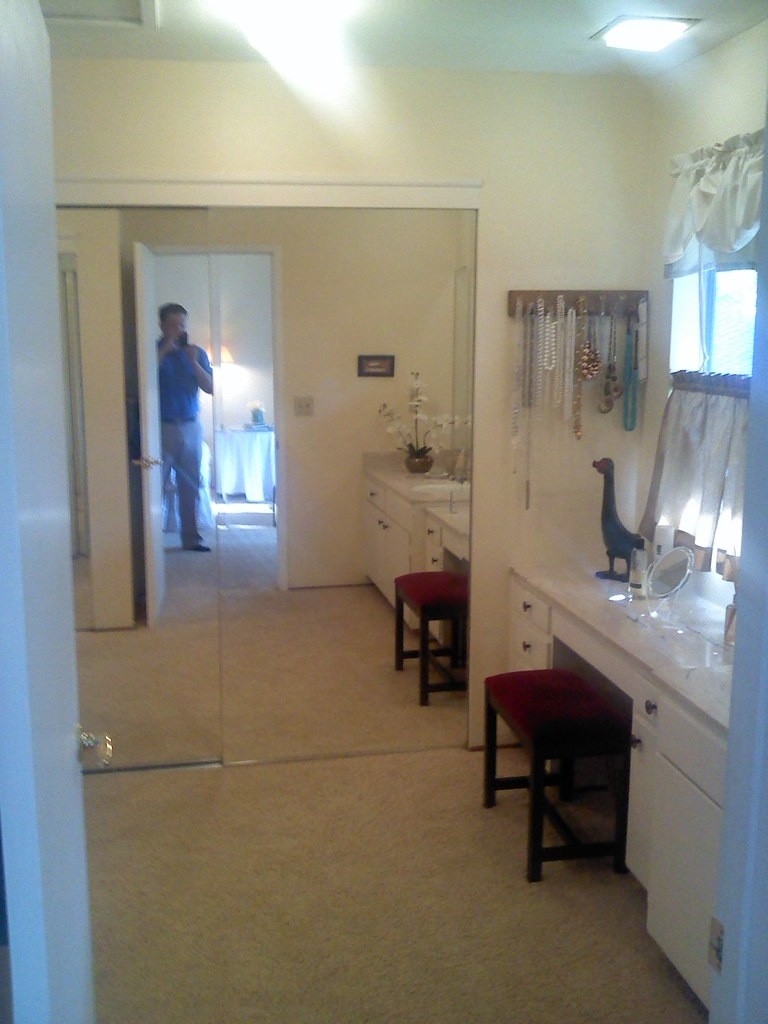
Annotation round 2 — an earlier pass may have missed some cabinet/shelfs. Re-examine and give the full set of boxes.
[361,469,472,628]
[423,506,470,643]
[645,664,733,1009]
[508,565,736,888]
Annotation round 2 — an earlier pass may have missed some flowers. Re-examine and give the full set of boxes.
[377,371,472,455]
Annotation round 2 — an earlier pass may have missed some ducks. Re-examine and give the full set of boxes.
[593,458,646,583]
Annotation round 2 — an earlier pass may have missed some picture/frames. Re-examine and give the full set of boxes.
[357,355,395,378]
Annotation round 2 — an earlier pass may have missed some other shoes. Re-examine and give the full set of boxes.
[190,544,210,552]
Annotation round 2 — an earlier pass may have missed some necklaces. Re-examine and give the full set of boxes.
[512,293,650,508]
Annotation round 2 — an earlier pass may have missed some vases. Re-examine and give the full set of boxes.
[404,455,432,473]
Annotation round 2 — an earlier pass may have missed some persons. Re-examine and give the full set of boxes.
[158,303,214,552]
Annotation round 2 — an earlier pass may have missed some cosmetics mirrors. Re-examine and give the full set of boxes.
[646,545,694,599]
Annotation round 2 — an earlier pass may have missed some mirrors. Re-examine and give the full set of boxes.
[642,547,694,622]
[57,207,478,769]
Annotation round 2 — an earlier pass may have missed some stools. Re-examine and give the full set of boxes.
[481,667,631,883]
[393,572,471,706]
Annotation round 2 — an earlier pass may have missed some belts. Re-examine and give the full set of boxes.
[162,416,198,423]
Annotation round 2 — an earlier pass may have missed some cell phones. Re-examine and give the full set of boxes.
[178,332,188,352]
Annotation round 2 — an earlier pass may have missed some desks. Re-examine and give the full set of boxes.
[215,428,275,504]
